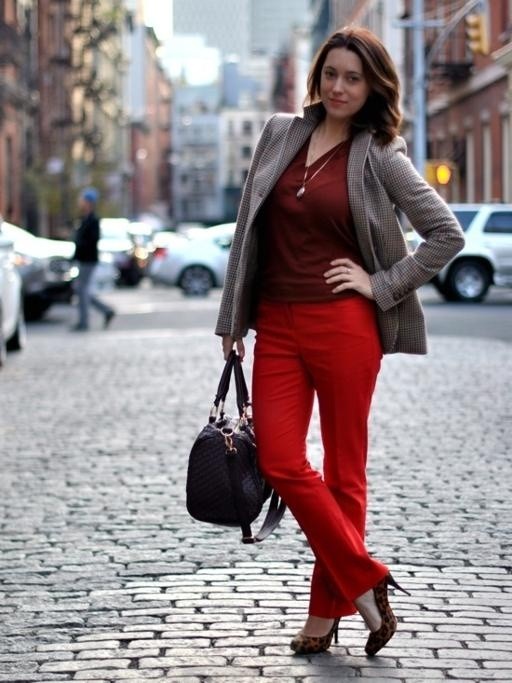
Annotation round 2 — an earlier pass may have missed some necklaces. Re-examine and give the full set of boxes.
[296,125,343,199]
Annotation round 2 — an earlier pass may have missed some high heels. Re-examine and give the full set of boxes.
[290,616,340,655]
[364,570,411,658]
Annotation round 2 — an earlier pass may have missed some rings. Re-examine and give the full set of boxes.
[347,267,351,274]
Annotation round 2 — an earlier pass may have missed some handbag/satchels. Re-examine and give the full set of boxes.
[185,416,272,528]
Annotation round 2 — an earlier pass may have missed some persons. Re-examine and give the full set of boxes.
[215,22,468,658]
[65,185,115,331]
